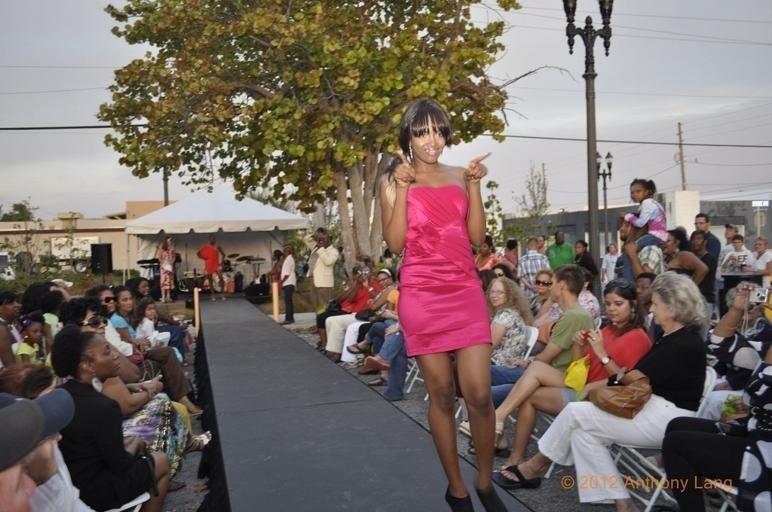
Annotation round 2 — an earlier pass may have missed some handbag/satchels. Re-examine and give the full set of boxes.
[588,369,652,418]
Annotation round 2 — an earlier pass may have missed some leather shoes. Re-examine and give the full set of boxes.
[446,485,474,511]
[368,377,386,385]
[360,366,378,374]
[168,479,185,491]
[459,418,504,447]
[476,484,508,511]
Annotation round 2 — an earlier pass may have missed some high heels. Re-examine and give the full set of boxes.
[183,430,212,460]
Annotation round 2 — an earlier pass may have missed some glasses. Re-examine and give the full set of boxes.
[78,315,108,328]
[100,296,119,303]
[535,279,552,286]
[606,280,630,289]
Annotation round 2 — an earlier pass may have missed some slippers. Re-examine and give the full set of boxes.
[189,408,203,415]
[347,343,370,353]
[365,356,391,370]
[491,464,541,490]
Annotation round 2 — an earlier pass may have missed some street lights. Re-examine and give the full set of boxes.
[593,151,612,255]
[563,1,614,309]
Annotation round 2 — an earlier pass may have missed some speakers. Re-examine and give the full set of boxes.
[245,283,269,305]
[91,243,112,274]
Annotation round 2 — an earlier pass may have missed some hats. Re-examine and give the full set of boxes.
[0,388,74,473]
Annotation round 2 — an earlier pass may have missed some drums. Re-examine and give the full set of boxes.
[222,260,230,270]
[211,271,235,292]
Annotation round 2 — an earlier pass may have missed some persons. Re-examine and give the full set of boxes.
[200,236,226,301]
[269,227,407,403]
[451,178,772,512]
[379,97,506,511]
[158,236,176,302]
[0,278,214,508]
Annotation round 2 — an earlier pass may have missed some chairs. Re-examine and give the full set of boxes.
[402,316,772,512]
[103,491,151,512]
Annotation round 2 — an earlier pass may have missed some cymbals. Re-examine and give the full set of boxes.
[228,254,240,258]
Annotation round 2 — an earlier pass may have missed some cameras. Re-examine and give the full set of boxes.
[357,270,368,279]
[749,288,768,303]
